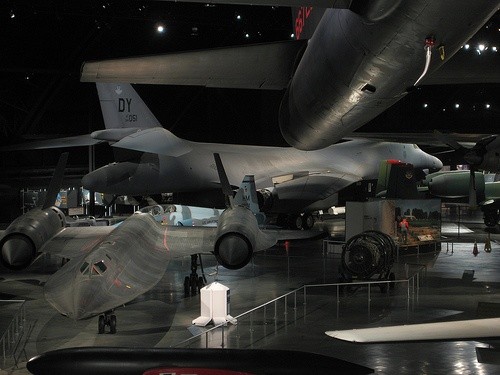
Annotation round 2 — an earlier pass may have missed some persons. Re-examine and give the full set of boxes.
[398,217,410,244]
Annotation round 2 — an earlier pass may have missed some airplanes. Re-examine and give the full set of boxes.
[1,1,497,344]
